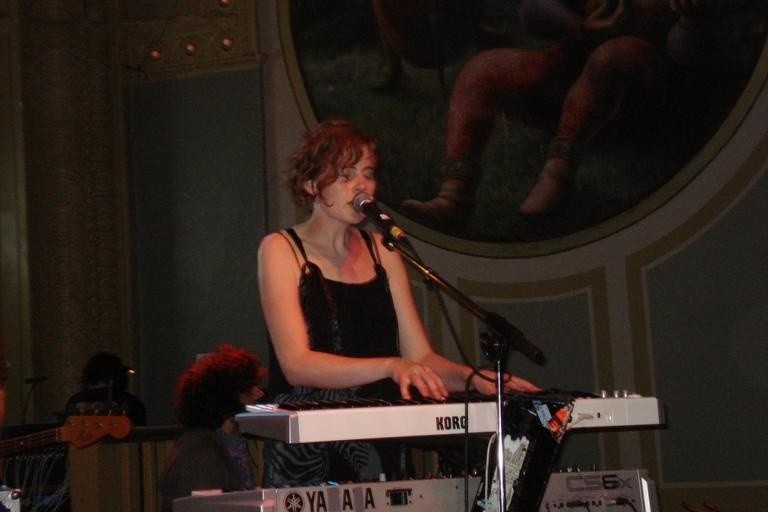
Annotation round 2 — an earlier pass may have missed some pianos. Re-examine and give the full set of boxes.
[172,390,669,512]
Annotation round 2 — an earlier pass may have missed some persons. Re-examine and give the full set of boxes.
[64,353,147,427]
[398,1,709,217]
[155,340,271,511]
[256,119,545,485]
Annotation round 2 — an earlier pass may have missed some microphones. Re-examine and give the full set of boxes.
[25,376,49,384]
[353,192,410,244]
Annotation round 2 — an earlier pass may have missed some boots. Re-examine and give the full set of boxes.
[515,134,591,224]
[399,155,481,235]
